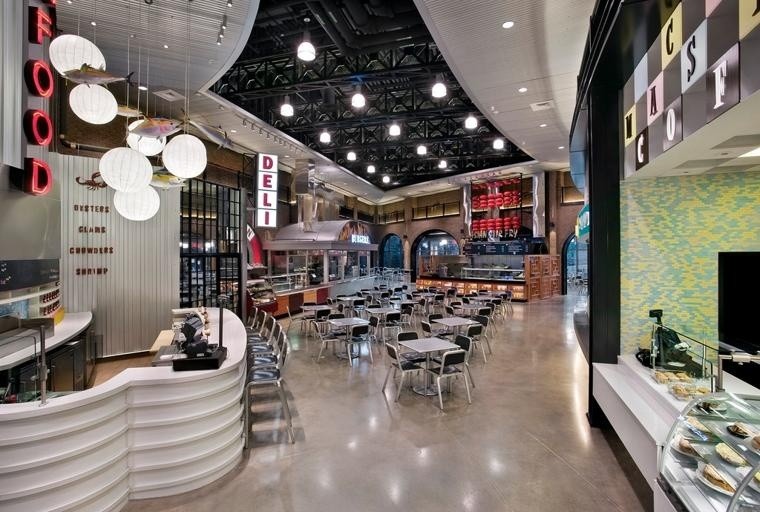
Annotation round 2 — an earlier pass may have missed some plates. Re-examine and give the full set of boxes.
[670,438,697,458]
[694,467,735,497]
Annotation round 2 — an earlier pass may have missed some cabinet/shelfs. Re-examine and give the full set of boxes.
[0,321,98,393]
[656,392,760,512]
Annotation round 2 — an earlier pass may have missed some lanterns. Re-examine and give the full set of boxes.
[472,179,520,231]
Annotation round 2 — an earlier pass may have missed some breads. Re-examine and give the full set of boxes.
[679,416,760,492]
[656,372,709,398]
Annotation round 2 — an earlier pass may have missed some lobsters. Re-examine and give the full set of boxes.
[76,171,107,191]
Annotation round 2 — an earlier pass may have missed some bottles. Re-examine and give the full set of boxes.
[43,290,59,315]
[205,318,210,328]
[204,308,208,318]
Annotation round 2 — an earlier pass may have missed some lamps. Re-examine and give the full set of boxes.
[279,29,506,184]
[48,0,207,220]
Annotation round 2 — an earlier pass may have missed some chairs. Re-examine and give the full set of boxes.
[239,266,512,449]
